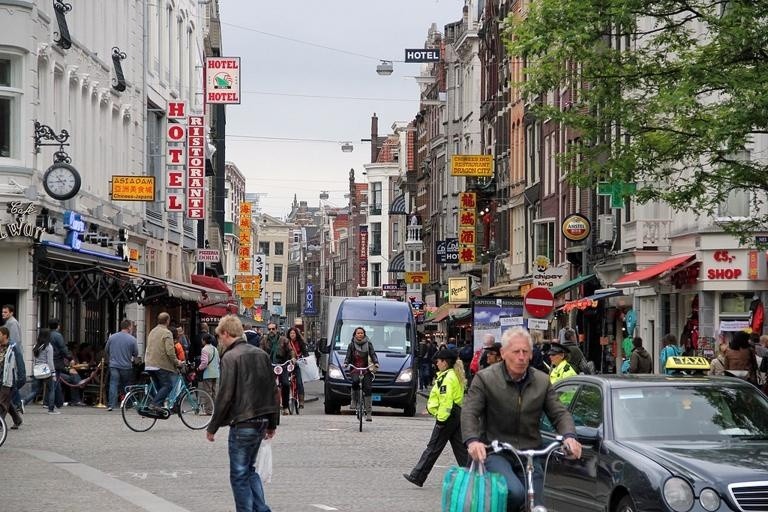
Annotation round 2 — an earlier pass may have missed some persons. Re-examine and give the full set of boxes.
[343,327,380,421]
[402,349,468,488]
[105,319,138,411]
[170,321,308,416]
[419,330,590,404]
[460,324,583,512]
[706,330,768,393]
[2,304,26,415]
[144,311,183,413]
[206,314,280,512]
[0,327,26,429]
[629,337,652,374]
[21,317,105,415]
[660,334,682,375]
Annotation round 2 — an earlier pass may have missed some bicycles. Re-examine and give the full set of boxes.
[468,432,591,512]
[0,415,7,448]
[120,361,215,433]
[341,359,379,432]
[287,357,304,416]
[271,359,292,425]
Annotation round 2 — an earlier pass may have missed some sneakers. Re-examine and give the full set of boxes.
[148,404,164,413]
[48,410,61,415]
[21,400,24,414]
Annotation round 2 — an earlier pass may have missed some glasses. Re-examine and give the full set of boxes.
[268,327,277,331]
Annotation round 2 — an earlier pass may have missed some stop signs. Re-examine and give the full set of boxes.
[524,286,556,318]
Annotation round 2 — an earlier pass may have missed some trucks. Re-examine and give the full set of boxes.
[313,295,391,382]
[318,298,421,416]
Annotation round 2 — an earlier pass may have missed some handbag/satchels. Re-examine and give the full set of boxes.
[367,355,377,373]
[440,458,508,511]
[33,364,52,379]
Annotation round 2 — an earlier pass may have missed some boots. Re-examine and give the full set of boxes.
[298,394,304,408]
[350,388,358,409]
[364,396,373,421]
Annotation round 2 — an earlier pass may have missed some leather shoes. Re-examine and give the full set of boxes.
[403,474,423,487]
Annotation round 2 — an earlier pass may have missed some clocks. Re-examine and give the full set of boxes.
[42,162,81,200]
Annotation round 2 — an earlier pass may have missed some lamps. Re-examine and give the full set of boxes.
[319,189,330,199]
[342,141,352,153]
[376,59,394,76]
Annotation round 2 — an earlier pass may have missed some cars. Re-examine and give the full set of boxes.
[528,356,767,512]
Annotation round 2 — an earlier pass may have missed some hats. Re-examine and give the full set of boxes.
[484,343,501,354]
[547,342,571,355]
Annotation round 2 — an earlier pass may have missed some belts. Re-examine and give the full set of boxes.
[231,422,268,429]
